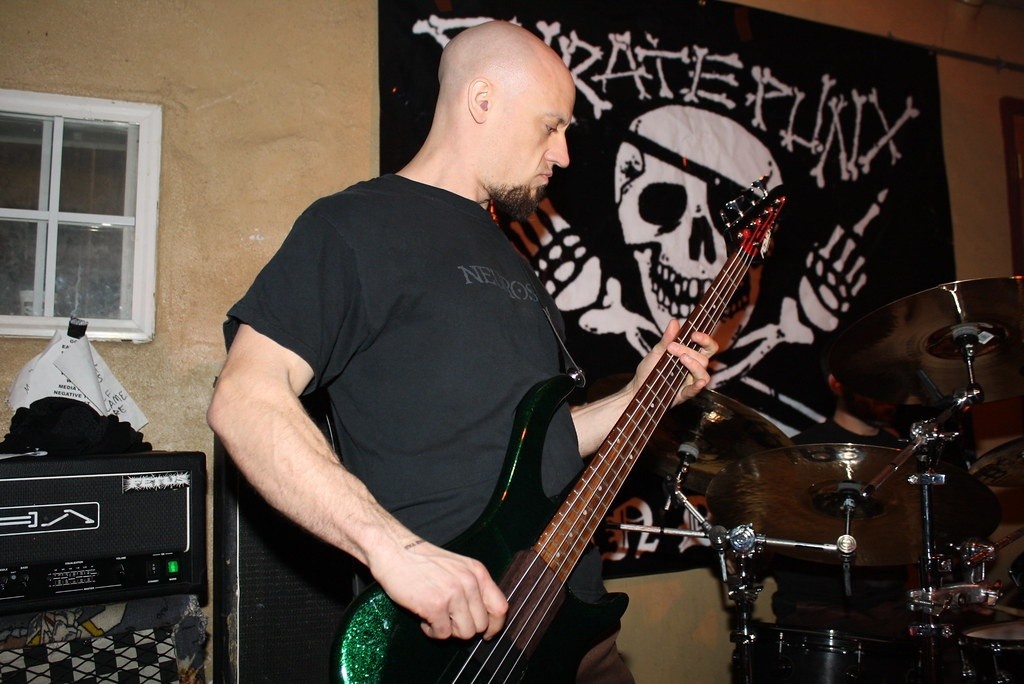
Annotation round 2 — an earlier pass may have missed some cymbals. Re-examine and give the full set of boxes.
[968,434,1024,489]
[645,385,796,476]
[705,442,1002,569]
[832,275,1023,407]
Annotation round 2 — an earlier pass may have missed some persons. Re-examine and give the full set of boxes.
[208,21,719,684]
[773,323,924,640]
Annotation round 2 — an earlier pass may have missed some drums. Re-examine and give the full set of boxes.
[732,620,931,684]
[957,616,1024,684]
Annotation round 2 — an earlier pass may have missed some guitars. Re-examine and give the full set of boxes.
[326,177,788,684]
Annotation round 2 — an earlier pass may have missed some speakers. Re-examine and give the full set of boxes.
[212,434,360,684]
